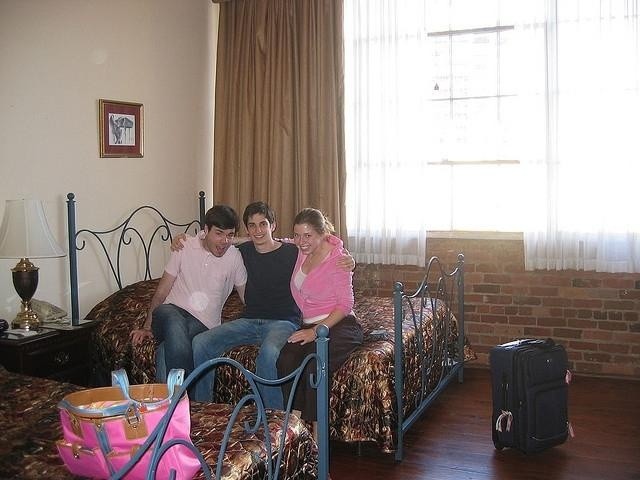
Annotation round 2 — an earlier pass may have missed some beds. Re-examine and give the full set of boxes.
[0,325,331,480]
[66,192,465,462]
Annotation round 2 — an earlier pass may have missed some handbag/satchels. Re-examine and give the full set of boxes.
[55,368,202,480]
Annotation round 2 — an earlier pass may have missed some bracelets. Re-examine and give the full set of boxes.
[312,327,317,338]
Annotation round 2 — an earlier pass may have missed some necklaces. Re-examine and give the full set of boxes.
[309,256,312,265]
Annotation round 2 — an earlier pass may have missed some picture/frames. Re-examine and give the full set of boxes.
[99,99,144,158]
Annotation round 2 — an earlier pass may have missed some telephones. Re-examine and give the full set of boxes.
[20,298,67,320]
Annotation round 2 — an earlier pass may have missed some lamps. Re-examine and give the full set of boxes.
[0,199,67,331]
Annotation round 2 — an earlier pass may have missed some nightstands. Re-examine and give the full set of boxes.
[0,320,101,387]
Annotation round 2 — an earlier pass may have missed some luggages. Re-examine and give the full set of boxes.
[488,336,571,456]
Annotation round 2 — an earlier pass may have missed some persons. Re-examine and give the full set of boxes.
[128,205,247,401]
[230,208,363,443]
[170,202,356,413]
